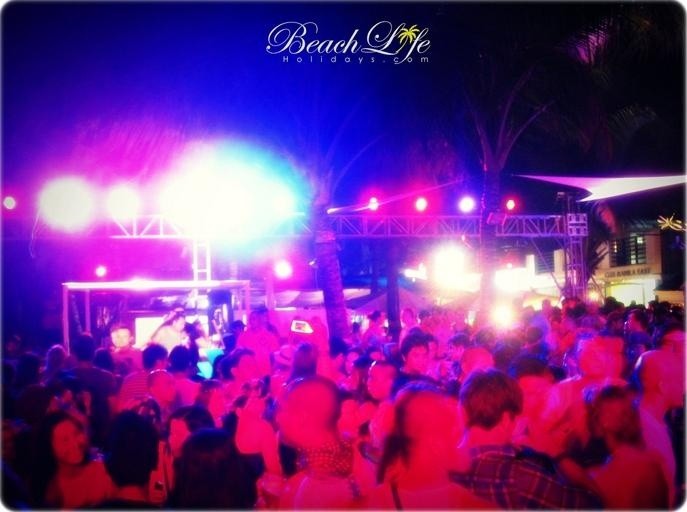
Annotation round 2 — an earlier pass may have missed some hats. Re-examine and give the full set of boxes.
[273,344,296,367]
